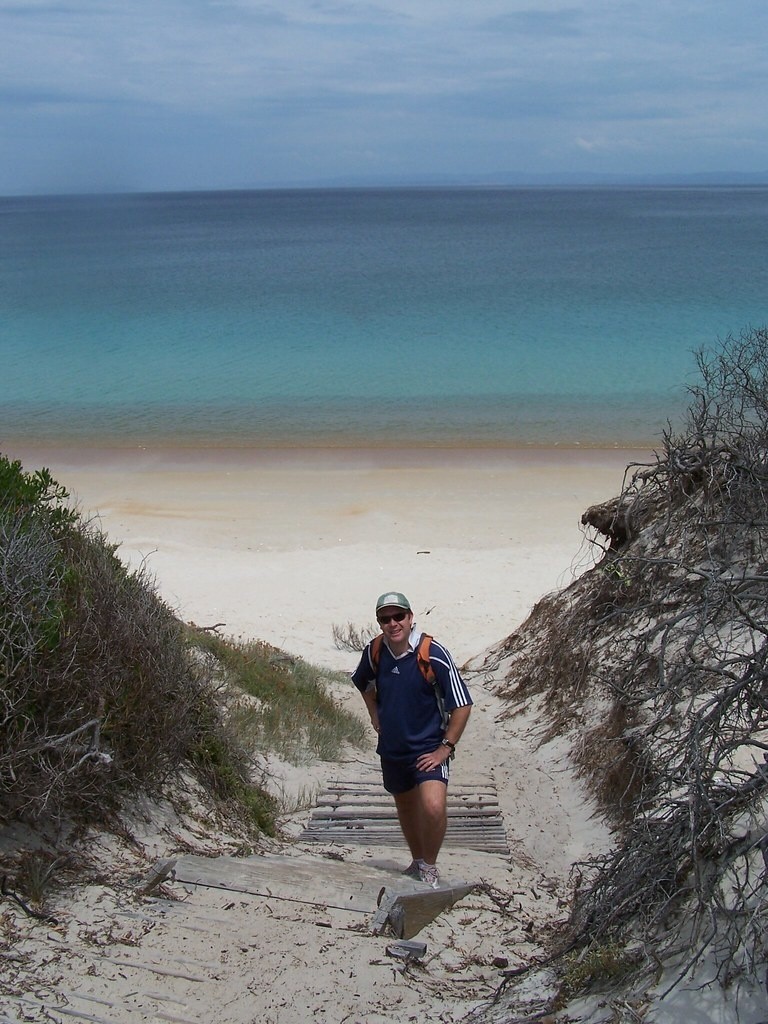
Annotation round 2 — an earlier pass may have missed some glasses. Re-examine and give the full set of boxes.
[377,613,406,624]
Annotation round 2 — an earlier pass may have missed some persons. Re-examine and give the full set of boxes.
[350,592,473,890]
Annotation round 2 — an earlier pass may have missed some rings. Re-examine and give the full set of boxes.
[429,761,432,764]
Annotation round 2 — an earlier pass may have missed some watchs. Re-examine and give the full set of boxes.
[441,738,454,749]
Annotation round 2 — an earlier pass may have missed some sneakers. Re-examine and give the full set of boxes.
[401,861,419,877]
[418,864,441,890]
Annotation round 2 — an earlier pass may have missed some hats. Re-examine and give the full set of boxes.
[376,592,410,612]
